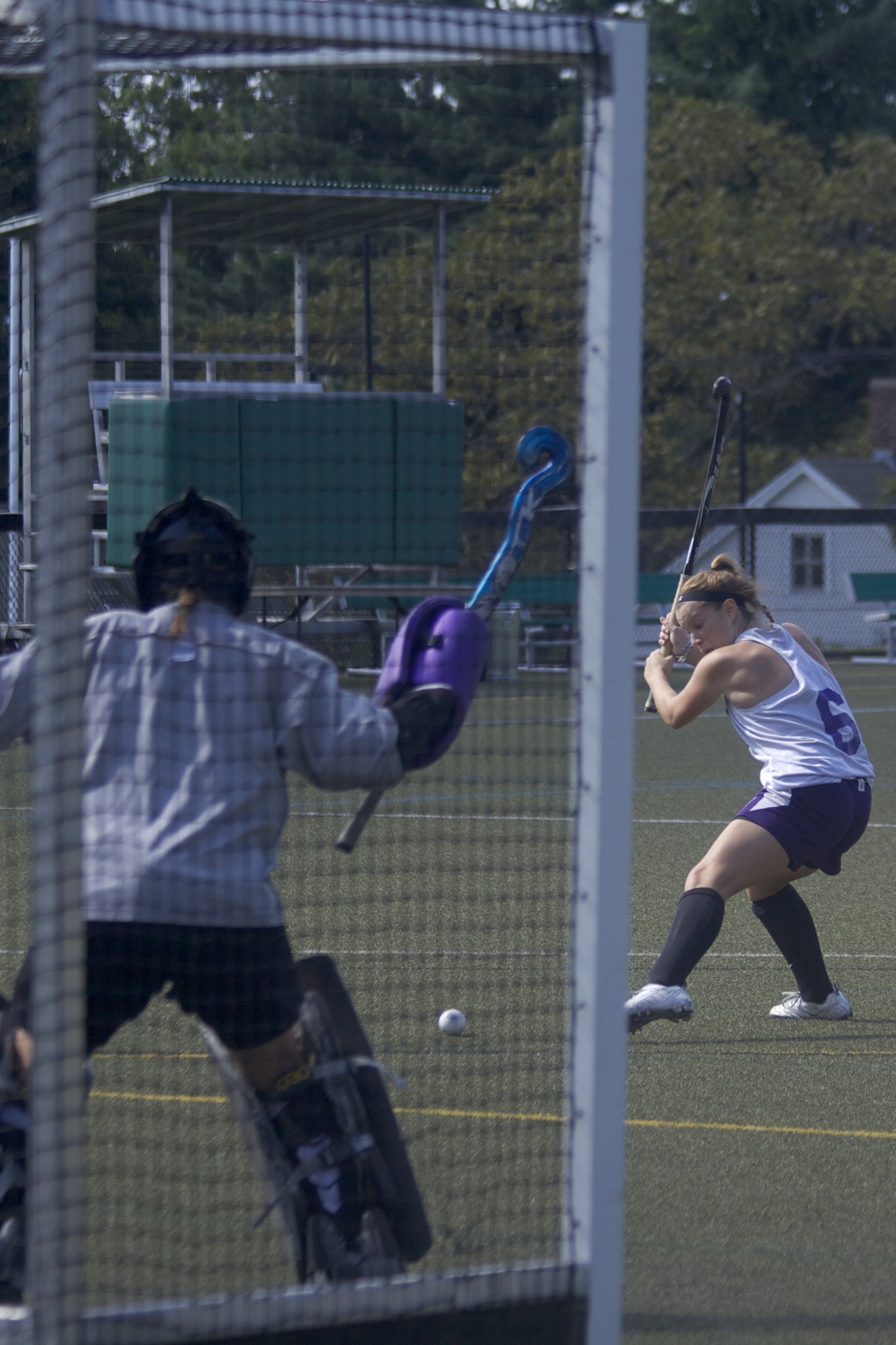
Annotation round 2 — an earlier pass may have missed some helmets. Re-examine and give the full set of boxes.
[133,485,253,619]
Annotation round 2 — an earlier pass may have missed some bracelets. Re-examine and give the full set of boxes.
[676,640,693,664]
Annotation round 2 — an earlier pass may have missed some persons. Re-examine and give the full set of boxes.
[626,552,875,1036]
[0,491,494,1307]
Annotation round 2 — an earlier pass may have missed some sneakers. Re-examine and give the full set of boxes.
[624,983,694,1034]
[768,983,854,1022]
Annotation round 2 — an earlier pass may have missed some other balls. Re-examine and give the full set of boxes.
[438,1008,466,1035]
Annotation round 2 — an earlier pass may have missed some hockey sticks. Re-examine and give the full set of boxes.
[641,375,734,715]
[334,426,576,856]
